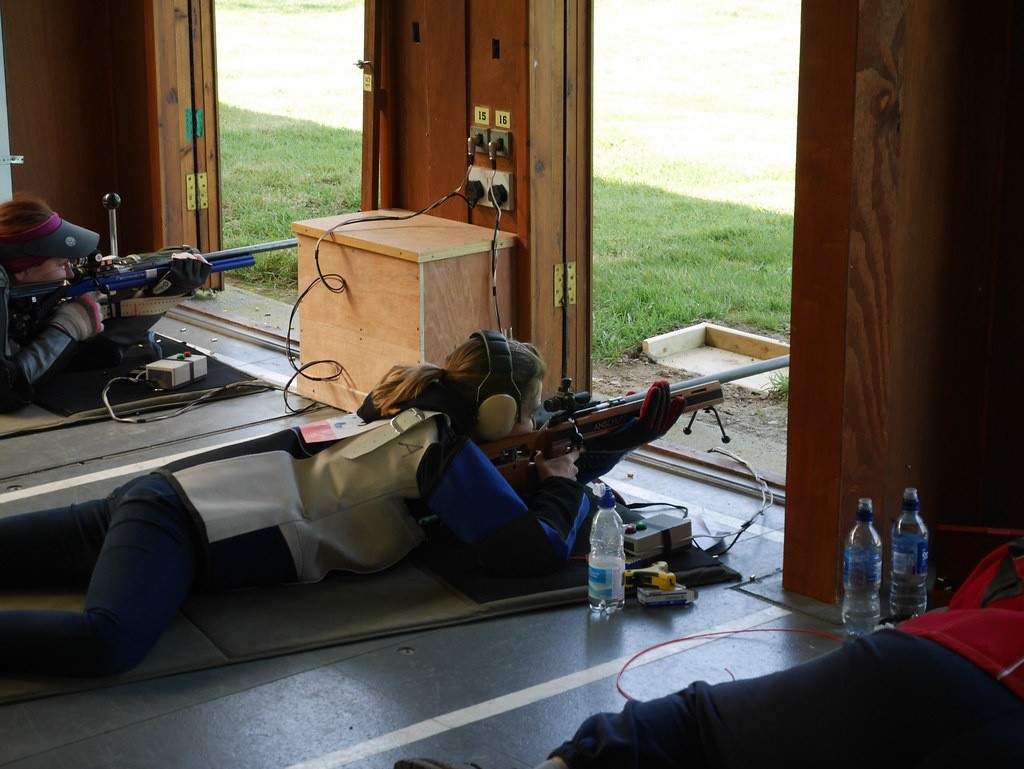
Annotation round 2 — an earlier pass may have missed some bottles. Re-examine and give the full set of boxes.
[587,488,625,614]
[888,487,930,619]
[841,498,883,635]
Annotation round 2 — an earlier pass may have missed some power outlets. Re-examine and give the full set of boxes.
[466,165,513,211]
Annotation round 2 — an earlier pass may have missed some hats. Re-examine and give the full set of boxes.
[0,212,100,274]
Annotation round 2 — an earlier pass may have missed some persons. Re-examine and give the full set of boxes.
[0,329,688,680]
[0,198,212,414]
[393,540,1024,769]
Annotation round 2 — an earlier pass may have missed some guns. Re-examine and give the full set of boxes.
[5,237,299,311]
[476,353,791,497]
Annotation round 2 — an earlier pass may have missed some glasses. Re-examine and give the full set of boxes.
[28,259,70,275]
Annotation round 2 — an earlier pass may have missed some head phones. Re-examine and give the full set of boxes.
[468,330,522,439]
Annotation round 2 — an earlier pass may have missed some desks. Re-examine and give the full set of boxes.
[292,208,520,416]
